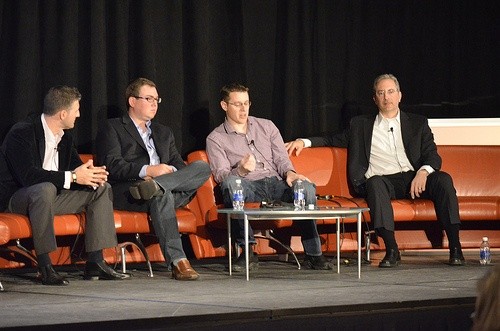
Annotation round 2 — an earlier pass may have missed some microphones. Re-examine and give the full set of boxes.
[58,147,61,152]
[391,127,393,132]
[251,140,254,144]
[149,134,153,139]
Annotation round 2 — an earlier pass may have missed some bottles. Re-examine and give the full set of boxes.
[293,178,306,208]
[232,179,244,210]
[479,237,491,264]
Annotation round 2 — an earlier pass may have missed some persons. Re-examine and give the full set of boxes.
[96,77,212,280]
[206,84,331,272]
[0,85,130,285]
[285,75,466,266]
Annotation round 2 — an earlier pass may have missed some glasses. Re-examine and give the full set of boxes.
[135,95,162,104]
[377,88,398,97]
[228,101,252,108]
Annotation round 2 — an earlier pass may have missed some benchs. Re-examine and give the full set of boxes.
[0,153,198,291]
[186,143,499,260]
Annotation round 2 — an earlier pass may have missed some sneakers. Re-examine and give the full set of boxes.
[303,256,334,271]
[232,252,260,272]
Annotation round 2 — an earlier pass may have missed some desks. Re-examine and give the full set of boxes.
[218,207,372,280]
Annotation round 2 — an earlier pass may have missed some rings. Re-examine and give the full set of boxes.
[418,188,422,189]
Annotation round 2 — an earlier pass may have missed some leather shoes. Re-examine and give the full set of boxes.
[36,264,69,285]
[83,259,132,281]
[129,177,158,200]
[172,259,200,280]
[379,248,401,268]
[449,245,465,265]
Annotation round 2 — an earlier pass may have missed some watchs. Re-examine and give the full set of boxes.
[72,171,77,182]
